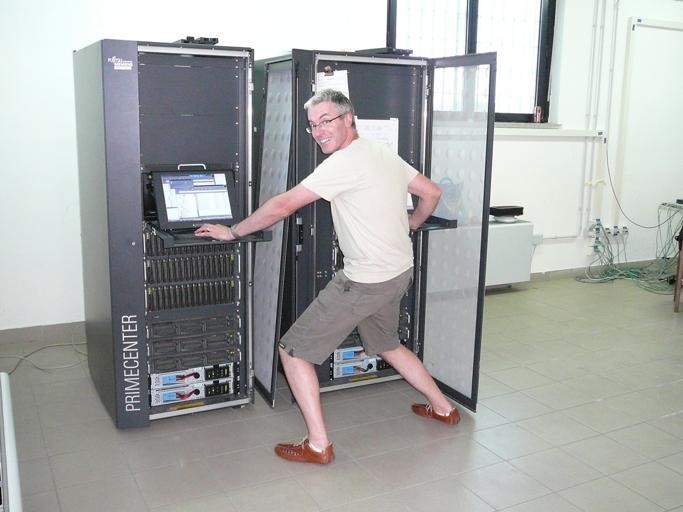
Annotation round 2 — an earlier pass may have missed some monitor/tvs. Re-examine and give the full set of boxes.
[151,171,239,231]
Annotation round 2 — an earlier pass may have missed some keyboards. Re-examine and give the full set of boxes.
[174,231,257,244]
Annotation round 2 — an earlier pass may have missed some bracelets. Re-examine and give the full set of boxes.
[230,224,240,239]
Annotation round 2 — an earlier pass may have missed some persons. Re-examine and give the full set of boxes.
[193,88,461,466]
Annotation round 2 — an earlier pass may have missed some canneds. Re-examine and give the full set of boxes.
[534,106,542,123]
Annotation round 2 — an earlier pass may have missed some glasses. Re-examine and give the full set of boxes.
[304,114,344,135]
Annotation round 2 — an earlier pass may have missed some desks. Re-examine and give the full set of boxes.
[660,198,681,313]
[426,218,534,295]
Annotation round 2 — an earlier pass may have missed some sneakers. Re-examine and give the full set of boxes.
[274,435,336,466]
[411,400,461,426]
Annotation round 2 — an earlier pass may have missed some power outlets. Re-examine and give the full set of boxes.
[587,218,630,258]
